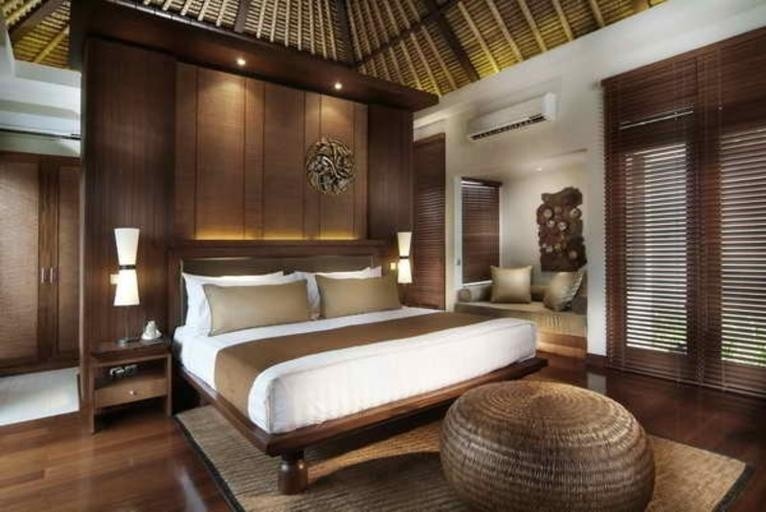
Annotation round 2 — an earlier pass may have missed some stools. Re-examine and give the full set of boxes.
[437,377,657,510]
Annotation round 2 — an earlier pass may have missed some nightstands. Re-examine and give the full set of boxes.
[81,333,174,435]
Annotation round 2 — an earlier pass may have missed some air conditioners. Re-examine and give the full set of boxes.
[466,91,557,142]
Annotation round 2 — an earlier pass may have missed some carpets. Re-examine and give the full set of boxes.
[170,398,752,512]
[0,362,82,428]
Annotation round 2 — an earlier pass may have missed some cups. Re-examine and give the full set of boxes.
[145,320,156,336]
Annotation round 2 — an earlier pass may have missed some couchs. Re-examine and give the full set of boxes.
[454,280,587,360]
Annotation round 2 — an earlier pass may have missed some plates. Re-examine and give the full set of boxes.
[140,331,163,341]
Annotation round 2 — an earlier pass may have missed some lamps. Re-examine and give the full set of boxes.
[389,231,414,285]
[107,226,140,307]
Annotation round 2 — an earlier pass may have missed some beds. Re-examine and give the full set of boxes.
[161,235,549,496]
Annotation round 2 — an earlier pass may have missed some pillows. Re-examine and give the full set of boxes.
[177,264,402,337]
[489,264,534,304]
[542,269,586,312]
